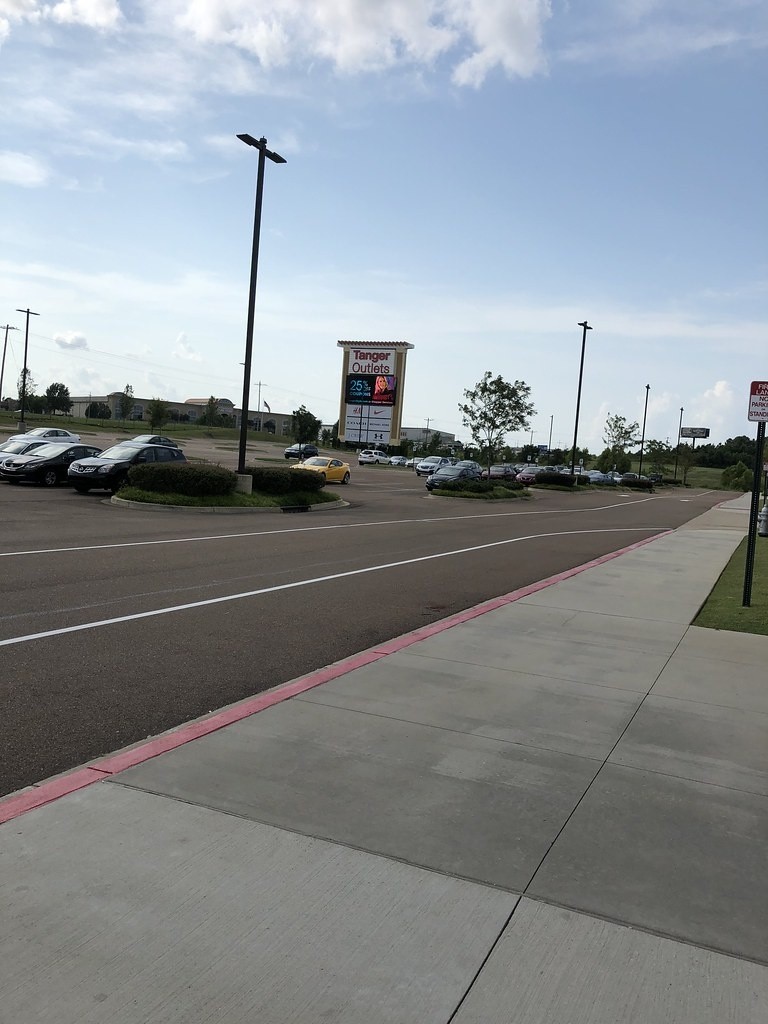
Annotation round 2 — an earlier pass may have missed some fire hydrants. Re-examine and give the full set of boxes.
[757,504,768,536]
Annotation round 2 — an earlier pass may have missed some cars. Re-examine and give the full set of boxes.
[482,465,517,481]
[391,456,409,467]
[15,410,29,414]
[0,439,54,463]
[130,435,178,448]
[289,456,351,484]
[447,457,460,465]
[0,442,105,487]
[8,426,81,444]
[455,460,483,475]
[514,464,650,485]
[426,465,480,490]
[515,466,548,486]
[404,457,423,467]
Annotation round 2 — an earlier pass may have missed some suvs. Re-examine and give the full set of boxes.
[415,455,452,476]
[284,443,319,460]
[358,450,392,467]
[67,440,189,495]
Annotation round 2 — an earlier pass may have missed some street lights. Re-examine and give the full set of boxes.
[548,415,553,458]
[16,308,41,422]
[572,321,593,477]
[639,384,650,480]
[255,384,267,430]
[675,407,684,479]
[235,132,286,474]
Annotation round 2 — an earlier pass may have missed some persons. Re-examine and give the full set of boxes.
[374,376,388,393]
